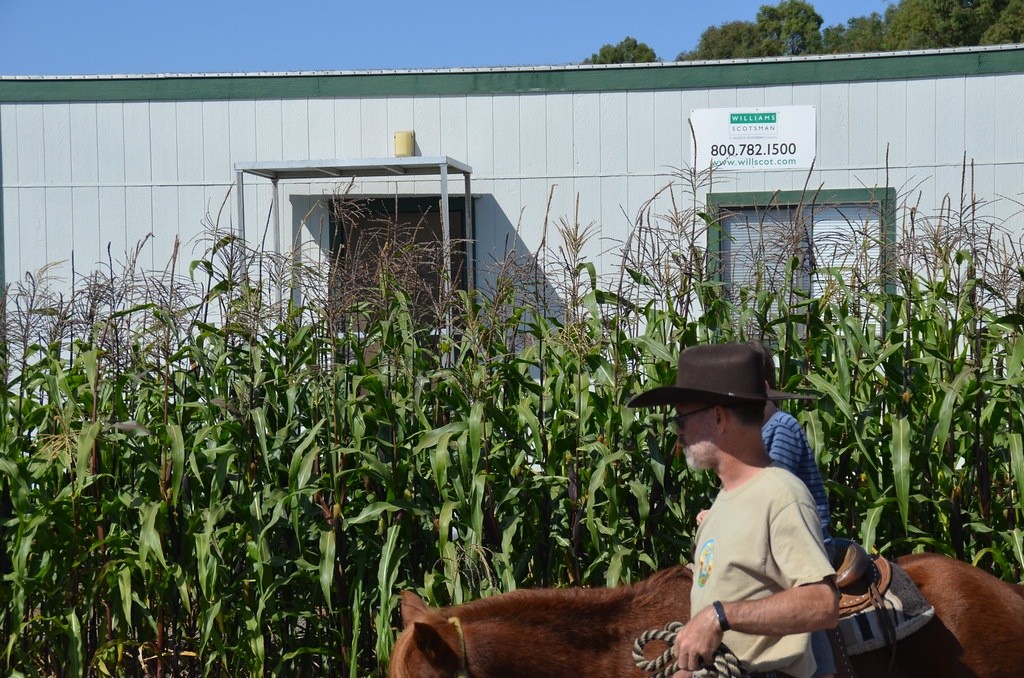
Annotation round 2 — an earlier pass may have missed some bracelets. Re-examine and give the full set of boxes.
[712,600,730,632]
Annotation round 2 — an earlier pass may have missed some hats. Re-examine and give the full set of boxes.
[627,344,818,407]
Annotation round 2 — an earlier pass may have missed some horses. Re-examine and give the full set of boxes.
[386,550,1024,678]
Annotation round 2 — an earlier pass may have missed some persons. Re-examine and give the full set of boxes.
[696,341,838,678]
[628,343,840,678]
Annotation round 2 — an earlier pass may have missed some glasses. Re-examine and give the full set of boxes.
[671,406,711,428]
